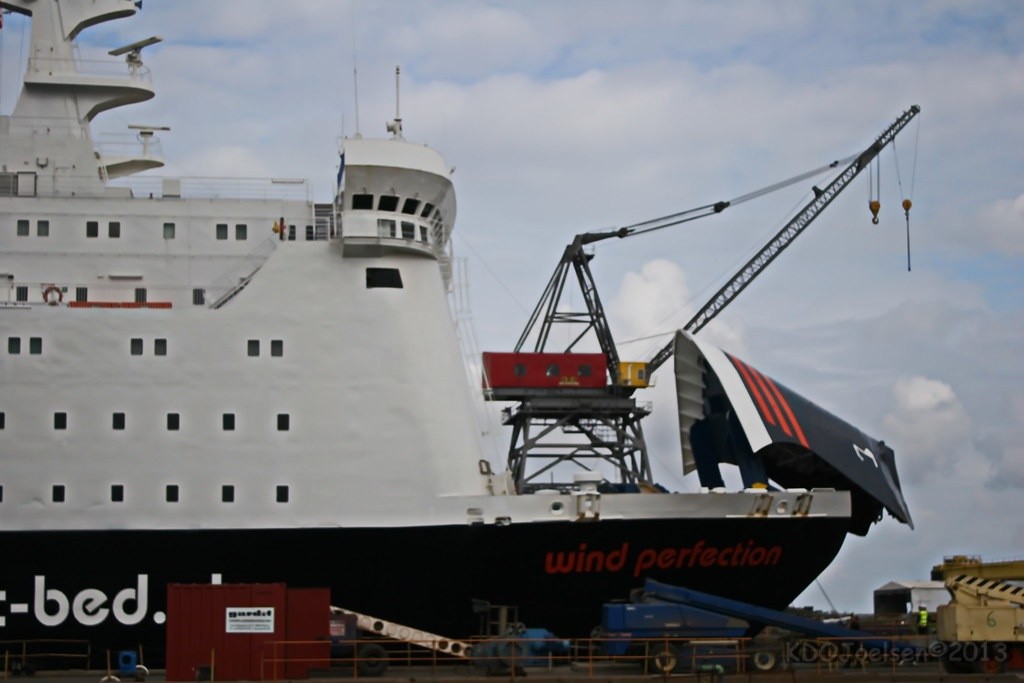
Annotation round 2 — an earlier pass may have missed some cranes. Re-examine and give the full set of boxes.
[482,86,923,499]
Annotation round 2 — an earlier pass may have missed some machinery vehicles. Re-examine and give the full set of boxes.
[923,554,1024,673]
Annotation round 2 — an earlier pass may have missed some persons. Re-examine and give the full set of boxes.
[917,604,928,635]
[846,614,860,630]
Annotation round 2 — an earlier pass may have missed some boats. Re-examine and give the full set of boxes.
[0,0,856,641]
